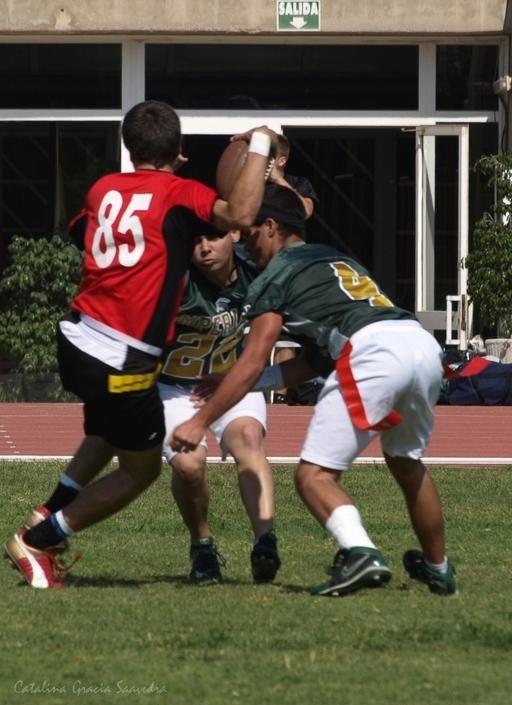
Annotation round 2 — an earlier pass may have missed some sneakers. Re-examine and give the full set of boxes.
[186,538,222,583]
[2,505,65,589]
[311,548,393,597]
[403,548,458,597]
[251,533,281,584]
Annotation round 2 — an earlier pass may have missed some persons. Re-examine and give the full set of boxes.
[269,134,320,403]
[170,187,456,598]
[157,223,280,584]
[3,100,279,590]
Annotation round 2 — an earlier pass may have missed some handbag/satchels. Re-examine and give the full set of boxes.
[438,344,512,404]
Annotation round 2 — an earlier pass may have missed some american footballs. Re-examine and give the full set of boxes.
[215,139,276,201]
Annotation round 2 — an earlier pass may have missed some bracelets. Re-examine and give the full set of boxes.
[248,130,271,157]
